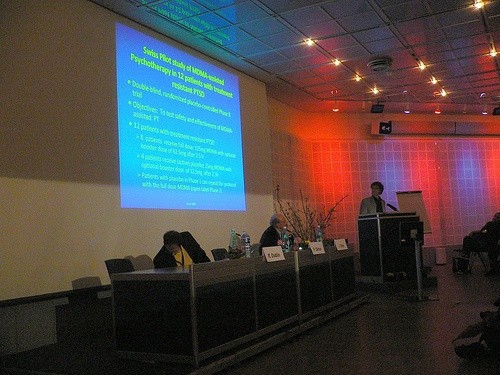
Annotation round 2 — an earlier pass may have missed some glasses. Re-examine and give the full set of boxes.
[164,248,180,254]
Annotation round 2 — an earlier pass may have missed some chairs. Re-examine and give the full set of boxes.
[211,249,227,261]
[105,259,135,278]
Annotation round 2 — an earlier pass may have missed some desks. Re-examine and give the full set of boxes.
[112,244,355,366]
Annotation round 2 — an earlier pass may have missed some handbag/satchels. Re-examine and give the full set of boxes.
[452,257,470,273]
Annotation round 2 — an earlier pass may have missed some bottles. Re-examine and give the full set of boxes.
[282,226,290,253]
[316,225,323,243]
[230,228,237,249]
[241,230,251,258]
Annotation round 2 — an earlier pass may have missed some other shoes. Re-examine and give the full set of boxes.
[455,342,485,358]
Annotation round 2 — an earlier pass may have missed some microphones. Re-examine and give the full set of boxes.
[387,203,397,211]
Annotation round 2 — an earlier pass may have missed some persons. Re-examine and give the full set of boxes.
[452,297,500,362]
[259,214,288,254]
[153,230,211,267]
[458,213,500,276]
[360,181,386,214]
